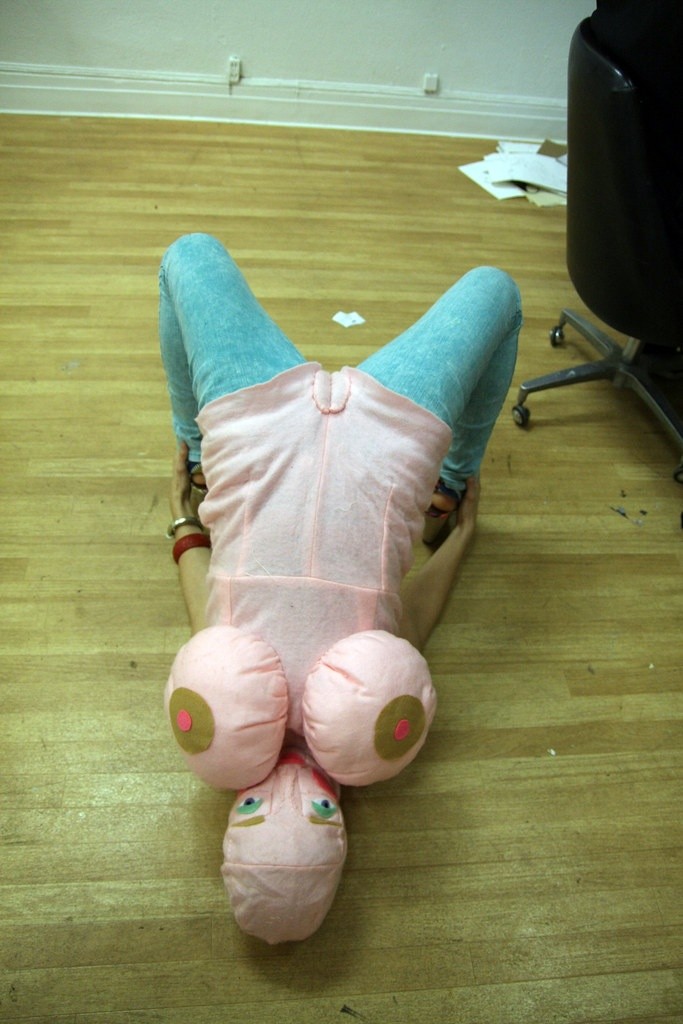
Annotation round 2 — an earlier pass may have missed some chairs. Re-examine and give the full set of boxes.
[511,0,683,482]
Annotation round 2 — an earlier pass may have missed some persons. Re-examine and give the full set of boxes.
[158,232,521,945]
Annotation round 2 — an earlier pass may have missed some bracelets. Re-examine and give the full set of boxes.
[166,517,204,537]
[173,533,210,564]
